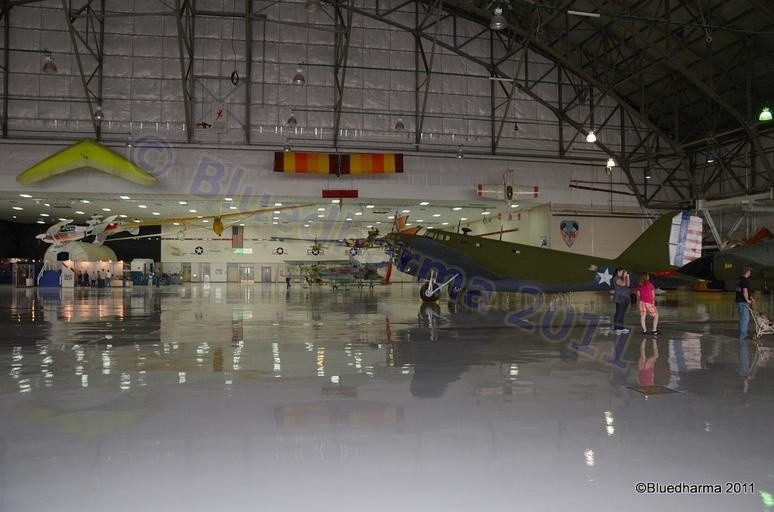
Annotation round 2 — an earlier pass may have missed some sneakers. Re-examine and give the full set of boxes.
[615,329,622,336]
[643,331,649,335]
[652,331,664,337]
[621,329,629,334]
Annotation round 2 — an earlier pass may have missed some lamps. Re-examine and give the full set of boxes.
[488,0,511,33]
[606,153,617,168]
[93,99,106,124]
[281,0,320,156]
[393,112,408,131]
[41,51,59,74]
[758,99,773,122]
[585,129,597,143]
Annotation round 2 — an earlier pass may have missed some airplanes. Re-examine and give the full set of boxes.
[34,198,774,319]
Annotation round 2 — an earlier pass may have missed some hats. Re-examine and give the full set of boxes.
[740,266,753,274]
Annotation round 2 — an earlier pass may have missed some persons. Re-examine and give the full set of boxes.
[608,336,759,397]
[612,265,631,337]
[147,269,153,287]
[734,264,755,340]
[77,268,111,288]
[635,270,665,335]
